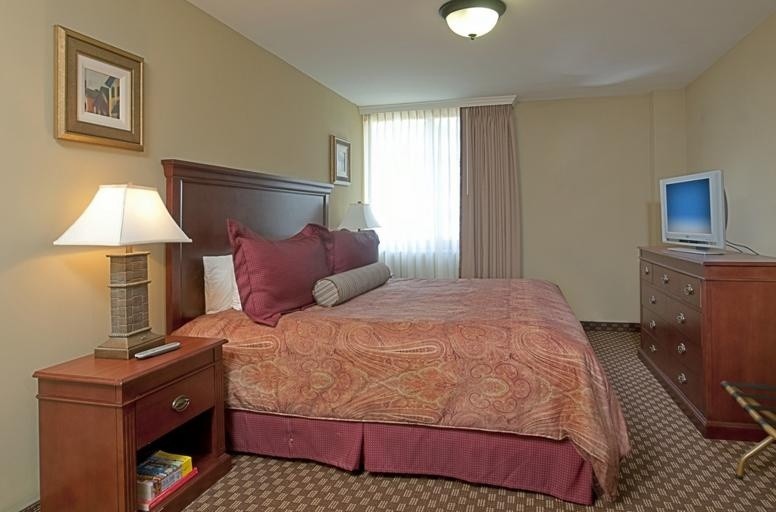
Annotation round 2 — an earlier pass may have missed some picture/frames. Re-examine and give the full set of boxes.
[330,134,352,186]
[53,24,144,151]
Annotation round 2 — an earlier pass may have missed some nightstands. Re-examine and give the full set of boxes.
[32,335,231,511]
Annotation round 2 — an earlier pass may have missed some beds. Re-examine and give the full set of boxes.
[161,158,632,507]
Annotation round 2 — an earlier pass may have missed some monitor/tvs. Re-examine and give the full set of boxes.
[658,169,727,254]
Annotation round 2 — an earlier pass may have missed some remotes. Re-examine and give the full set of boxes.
[134,342,181,359]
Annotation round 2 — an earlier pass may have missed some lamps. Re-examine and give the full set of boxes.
[336,201,379,232]
[52,182,193,360]
[438,1,506,41]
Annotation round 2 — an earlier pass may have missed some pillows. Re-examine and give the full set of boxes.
[202,218,391,327]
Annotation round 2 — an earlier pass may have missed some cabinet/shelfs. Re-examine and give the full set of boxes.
[637,245,775,442]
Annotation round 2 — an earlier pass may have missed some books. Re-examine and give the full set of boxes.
[135,449,199,510]
